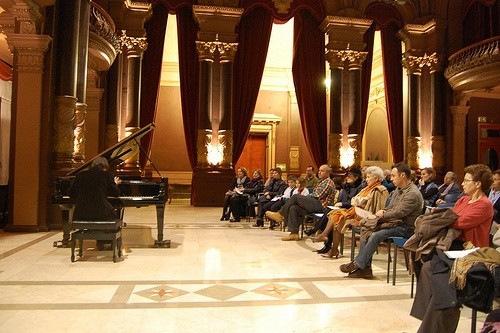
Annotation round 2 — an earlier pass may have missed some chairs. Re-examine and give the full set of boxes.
[248,190,500,333]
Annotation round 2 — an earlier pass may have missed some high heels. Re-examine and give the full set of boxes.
[323,248,339,258]
[252,219,264,227]
[310,234,328,243]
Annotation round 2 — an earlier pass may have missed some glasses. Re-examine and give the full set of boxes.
[463,179,475,182]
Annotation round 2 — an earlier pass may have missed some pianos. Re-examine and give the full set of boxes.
[47,122,171,248]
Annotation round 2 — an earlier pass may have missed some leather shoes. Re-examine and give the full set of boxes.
[304,229,315,236]
[319,245,331,253]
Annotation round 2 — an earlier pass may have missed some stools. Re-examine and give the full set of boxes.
[71,221,123,263]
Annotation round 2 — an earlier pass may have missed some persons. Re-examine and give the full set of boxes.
[68,156,120,251]
[219,164,500,333]
[340,163,424,279]
[412,164,495,285]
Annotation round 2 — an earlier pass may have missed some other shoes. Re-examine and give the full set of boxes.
[230,218,240,222]
[282,234,299,241]
[220,215,225,220]
[265,211,284,222]
[225,213,230,220]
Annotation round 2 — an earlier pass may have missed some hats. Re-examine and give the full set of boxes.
[348,168,360,176]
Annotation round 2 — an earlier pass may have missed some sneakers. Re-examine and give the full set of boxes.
[348,268,372,278]
[340,261,357,273]
